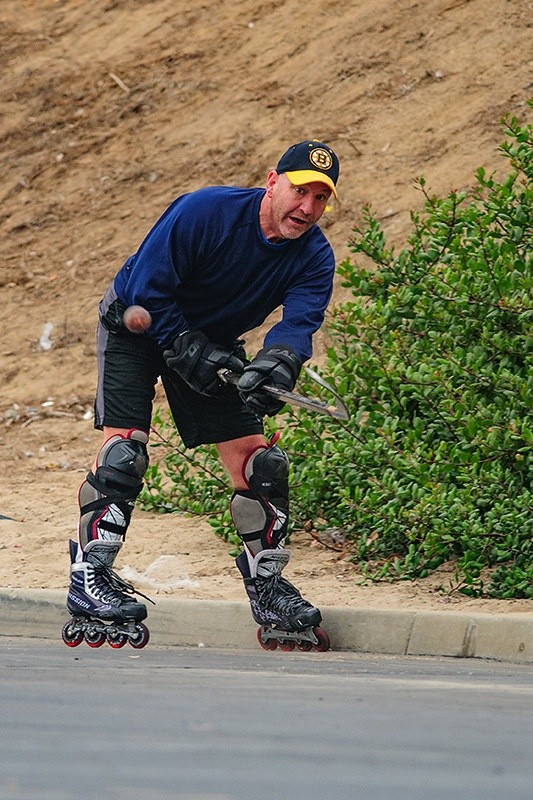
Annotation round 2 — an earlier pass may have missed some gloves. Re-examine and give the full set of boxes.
[237,343,302,418]
[162,327,251,399]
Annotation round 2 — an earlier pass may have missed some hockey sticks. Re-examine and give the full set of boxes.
[204,361,351,423]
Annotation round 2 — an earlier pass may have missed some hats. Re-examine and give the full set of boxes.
[275,138,340,200]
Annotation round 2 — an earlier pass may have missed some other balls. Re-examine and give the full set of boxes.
[122,305,151,333]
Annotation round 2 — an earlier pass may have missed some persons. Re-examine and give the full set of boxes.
[62,139,339,652]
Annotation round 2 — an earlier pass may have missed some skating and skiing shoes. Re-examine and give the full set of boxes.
[61,539,156,650]
[235,549,330,652]
